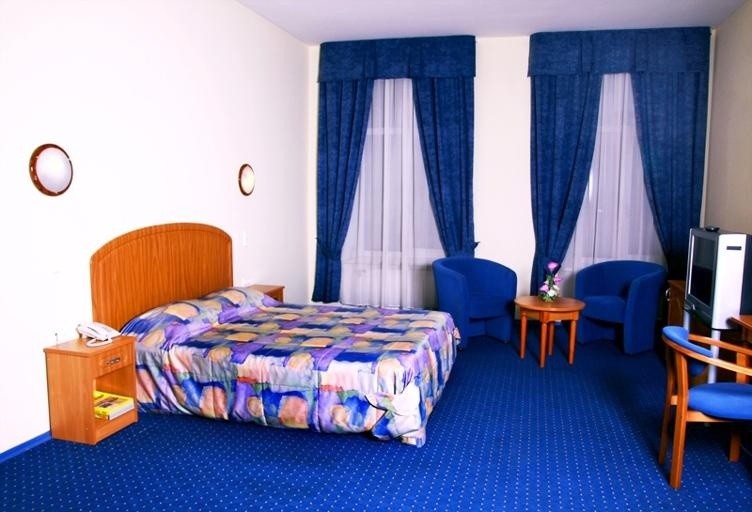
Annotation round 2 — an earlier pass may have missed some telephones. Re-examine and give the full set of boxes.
[77,322,121,341]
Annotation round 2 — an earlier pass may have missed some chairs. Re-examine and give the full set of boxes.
[431,254,515,348]
[654,322,752,492]
[574,260,669,358]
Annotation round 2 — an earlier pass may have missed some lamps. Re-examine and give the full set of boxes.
[237,163,255,194]
[29,143,73,196]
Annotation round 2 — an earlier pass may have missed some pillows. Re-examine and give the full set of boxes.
[122,283,275,349]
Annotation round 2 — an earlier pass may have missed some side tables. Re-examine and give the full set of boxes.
[512,295,586,368]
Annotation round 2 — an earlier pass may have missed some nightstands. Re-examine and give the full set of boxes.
[43,336,140,446]
[246,283,285,303]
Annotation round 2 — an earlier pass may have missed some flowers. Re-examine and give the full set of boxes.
[538,260,564,304]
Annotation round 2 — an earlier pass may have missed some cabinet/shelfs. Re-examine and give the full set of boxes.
[667,283,752,387]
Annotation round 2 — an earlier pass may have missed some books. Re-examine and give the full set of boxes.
[93,390,135,421]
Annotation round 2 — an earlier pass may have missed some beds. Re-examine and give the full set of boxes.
[93,224,455,446]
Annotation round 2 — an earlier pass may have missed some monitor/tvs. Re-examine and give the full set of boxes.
[684,227,752,329]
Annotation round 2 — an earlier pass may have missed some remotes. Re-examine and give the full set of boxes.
[705,224,720,232]
